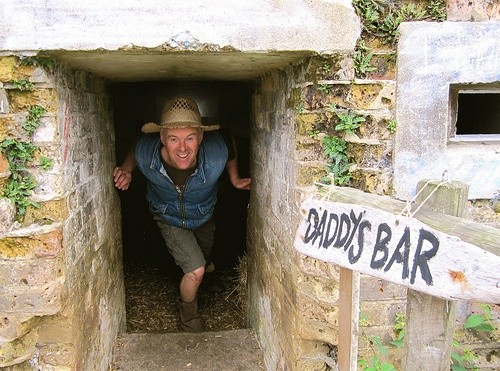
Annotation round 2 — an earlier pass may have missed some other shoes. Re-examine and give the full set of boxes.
[179,297,203,333]
[198,280,212,308]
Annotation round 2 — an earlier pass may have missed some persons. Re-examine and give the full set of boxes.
[112,97,251,331]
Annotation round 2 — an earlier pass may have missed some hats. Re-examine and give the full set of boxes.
[141,97,220,133]
